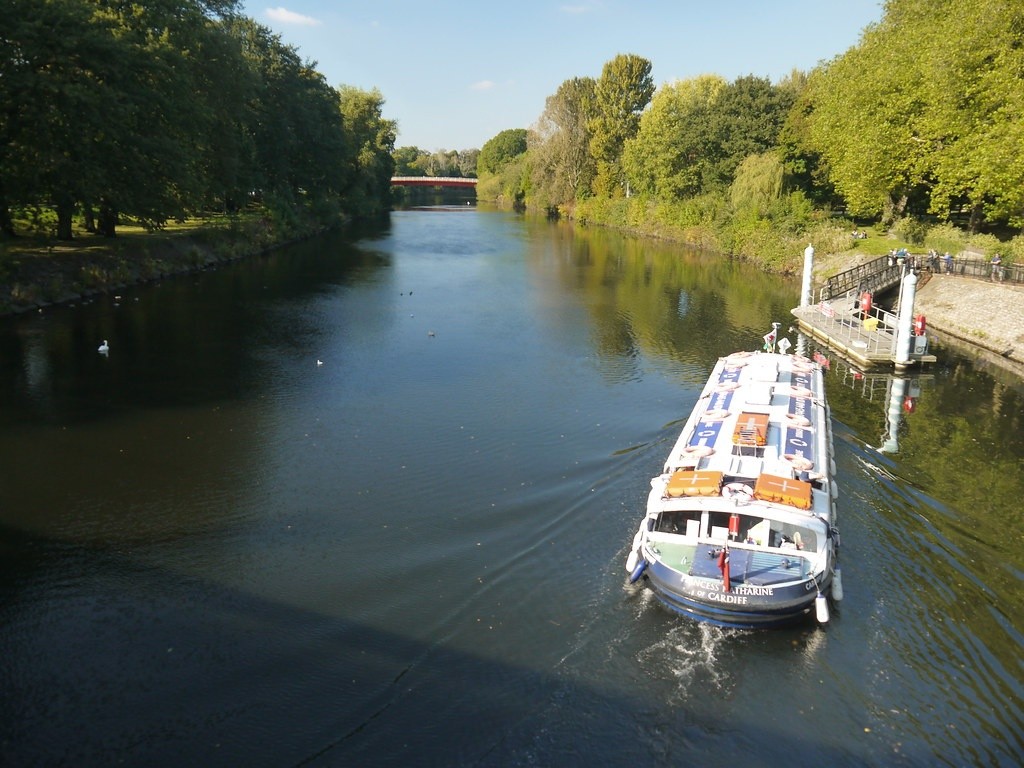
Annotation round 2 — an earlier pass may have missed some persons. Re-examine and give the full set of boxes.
[928,250,940,273]
[943,252,951,275]
[887,248,910,270]
[990,254,1001,281]
[861,230,866,238]
[850,228,858,238]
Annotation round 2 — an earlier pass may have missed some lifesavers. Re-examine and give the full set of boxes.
[682,444,714,457]
[711,381,738,392]
[724,361,746,370]
[791,362,812,372]
[778,453,814,470]
[701,409,728,420]
[782,385,813,397]
[727,352,751,359]
[783,412,811,427]
[721,482,755,505]
[791,355,810,363]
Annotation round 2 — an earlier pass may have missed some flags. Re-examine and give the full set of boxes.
[763,328,776,346]
[768,344,772,351]
[762,343,768,350]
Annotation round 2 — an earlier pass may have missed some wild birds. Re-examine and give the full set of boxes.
[98,339,109,352]
[317,360,323,365]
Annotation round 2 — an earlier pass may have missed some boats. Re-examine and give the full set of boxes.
[623,348,843,636]
